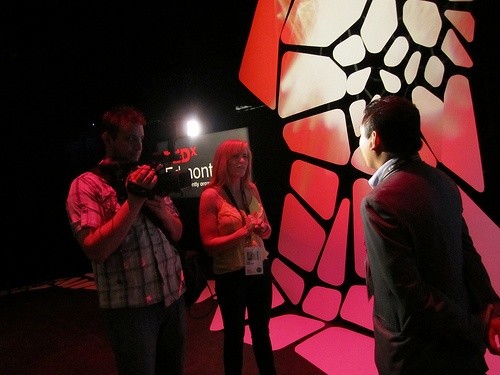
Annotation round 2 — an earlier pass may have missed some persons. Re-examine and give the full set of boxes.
[66,106,190,375]
[361,94,500,375]
[199,139,279,375]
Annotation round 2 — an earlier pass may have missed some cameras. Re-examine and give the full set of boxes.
[96,149,184,199]
